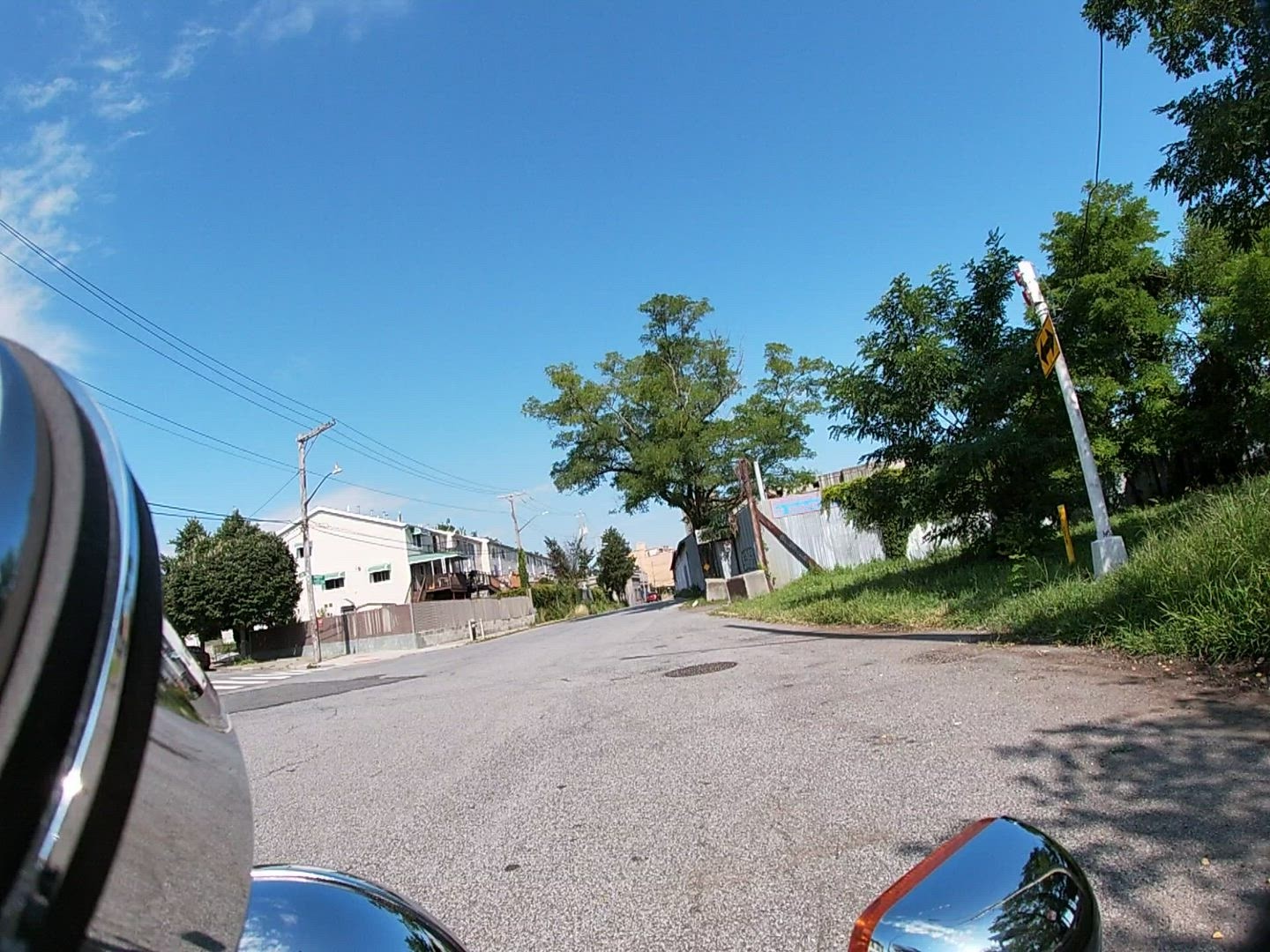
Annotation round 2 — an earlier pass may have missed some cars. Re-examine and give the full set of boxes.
[647,593,658,602]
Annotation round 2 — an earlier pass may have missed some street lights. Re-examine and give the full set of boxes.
[511,510,548,598]
[300,466,344,662]
